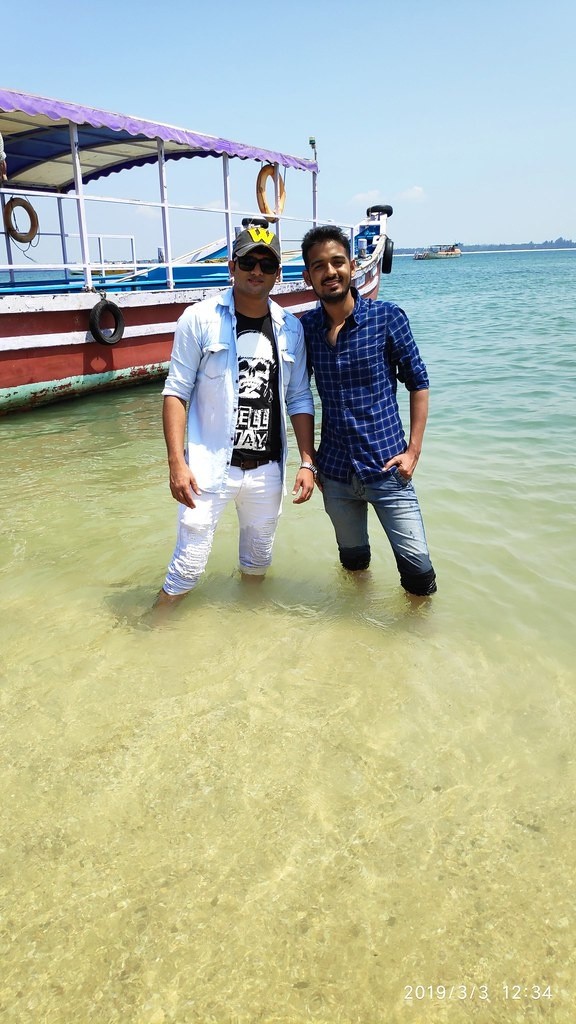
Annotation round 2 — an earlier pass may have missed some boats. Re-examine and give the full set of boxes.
[0,80,394,426]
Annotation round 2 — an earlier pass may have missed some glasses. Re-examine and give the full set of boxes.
[232,255,279,274]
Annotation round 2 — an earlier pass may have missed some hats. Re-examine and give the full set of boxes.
[232,227,281,262]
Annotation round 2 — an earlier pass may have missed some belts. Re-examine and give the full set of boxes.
[230,455,279,470]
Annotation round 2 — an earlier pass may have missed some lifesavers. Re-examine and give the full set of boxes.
[5,196,39,244]
[256,165,285,224]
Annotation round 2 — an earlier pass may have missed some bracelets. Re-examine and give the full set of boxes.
[300,461,319,481]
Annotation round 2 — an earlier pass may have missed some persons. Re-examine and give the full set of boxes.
[149,224,319,612]
[299,226,438,606]
